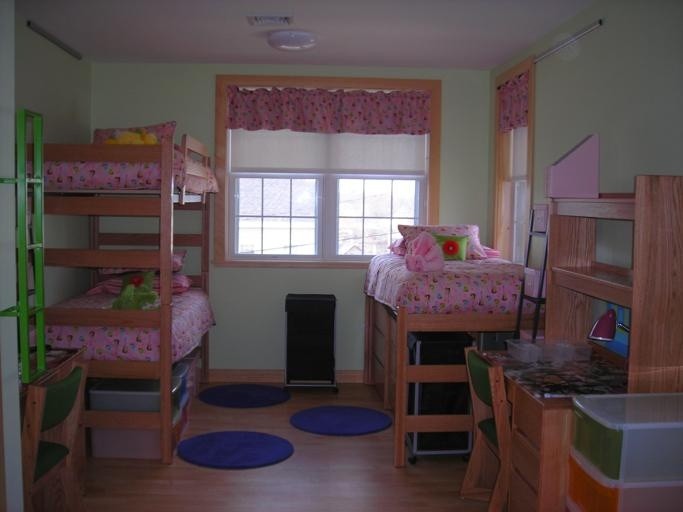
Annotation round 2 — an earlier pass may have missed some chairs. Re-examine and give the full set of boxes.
[26,348,88,512]
[457,349,512,511]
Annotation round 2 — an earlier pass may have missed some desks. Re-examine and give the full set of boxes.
[481,176,682,512]
[20,344,88,399]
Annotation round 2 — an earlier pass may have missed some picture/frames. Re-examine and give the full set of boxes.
[533,205,547,233]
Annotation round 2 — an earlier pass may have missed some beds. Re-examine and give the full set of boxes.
[26,133,212,465]
[367,255,538,467]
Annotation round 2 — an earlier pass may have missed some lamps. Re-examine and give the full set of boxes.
[533,19,602,65]
[587,310,629,345]
[28,20,82,60]
[266,30,319,52]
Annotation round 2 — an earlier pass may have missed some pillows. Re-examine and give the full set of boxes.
[93,120,177,143]
[88,276,191,294]
[96,250,187,274]
[388,225,499,260]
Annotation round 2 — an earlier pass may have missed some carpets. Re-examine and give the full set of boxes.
[197,384,290,409]
[179,431,294,469]
[290,406,392,435]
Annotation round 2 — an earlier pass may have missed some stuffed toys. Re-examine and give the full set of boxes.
[405,231,445,272]
[112,284,160,311]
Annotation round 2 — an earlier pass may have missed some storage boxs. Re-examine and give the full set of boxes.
[175,350,201,429]
[504,338,539,360]
[92,427,166,460]
[569,341,594,361]
[539,343,568,362]
[567,395,683,512]
[91,377,183,411]
[524,267,546,297]
